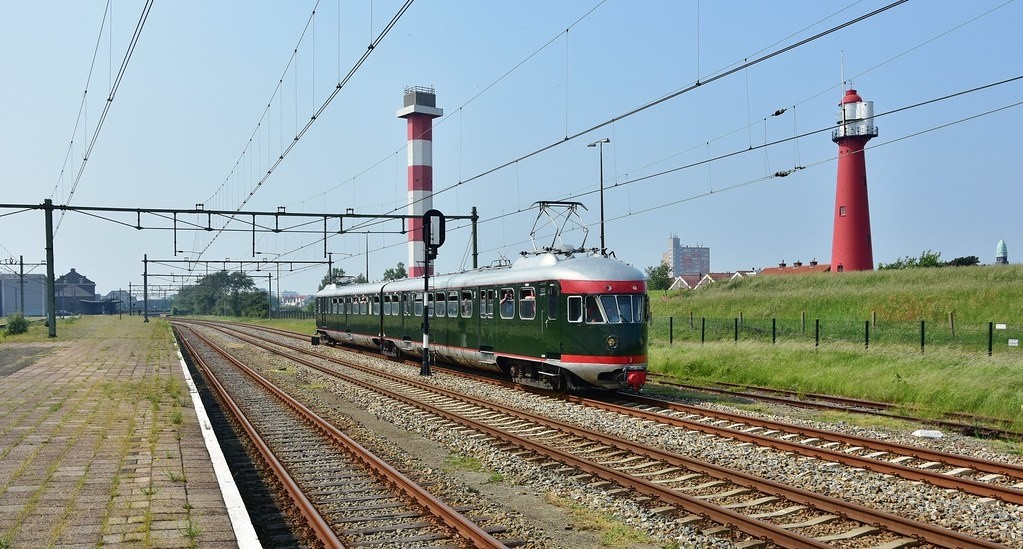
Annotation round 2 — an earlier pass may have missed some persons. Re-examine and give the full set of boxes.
[500,290,512,305]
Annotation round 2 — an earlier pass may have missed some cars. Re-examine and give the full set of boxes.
[55,310,72,316]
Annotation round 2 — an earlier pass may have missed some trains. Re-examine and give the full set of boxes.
[311,200,652,394]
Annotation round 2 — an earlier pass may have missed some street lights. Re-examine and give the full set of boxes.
[587,138,610,255]
